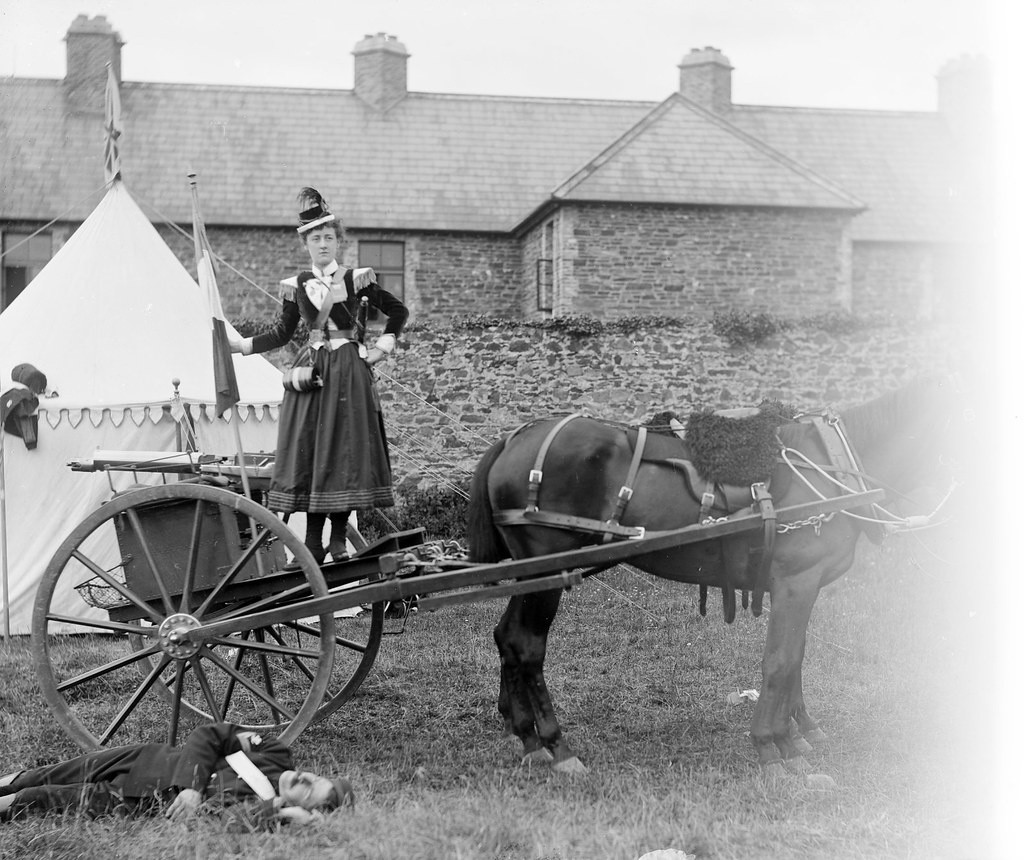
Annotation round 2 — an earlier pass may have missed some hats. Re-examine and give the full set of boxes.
[296,186,335,234]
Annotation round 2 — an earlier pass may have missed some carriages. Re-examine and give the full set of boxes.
[31,358,955,789]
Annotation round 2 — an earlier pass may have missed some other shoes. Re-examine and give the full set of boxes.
[282,545,327,571]
[329,541,350,562]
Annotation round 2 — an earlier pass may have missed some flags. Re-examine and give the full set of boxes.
[187,169,270,579]
[99,69,131,189]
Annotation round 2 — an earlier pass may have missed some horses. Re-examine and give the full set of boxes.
[468,377,949,772]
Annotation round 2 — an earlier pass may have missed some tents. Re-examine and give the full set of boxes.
[0,176,365,641]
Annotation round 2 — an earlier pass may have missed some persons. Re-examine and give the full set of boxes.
[0,719,357,832]
[224,186,409,572]
